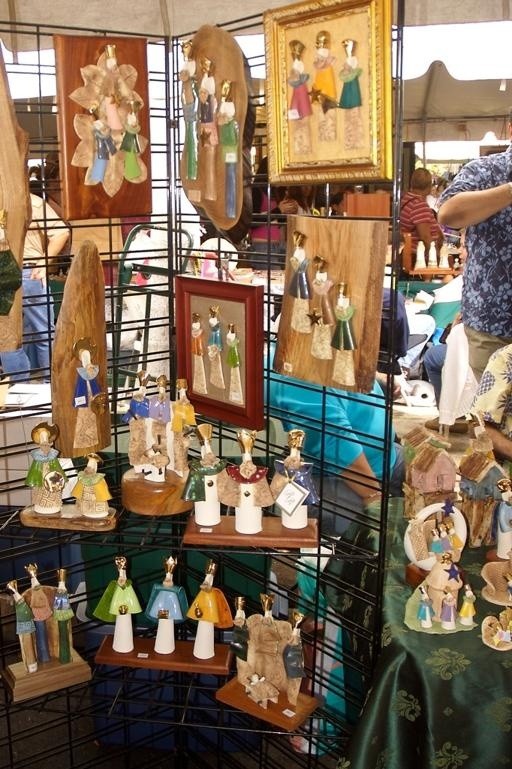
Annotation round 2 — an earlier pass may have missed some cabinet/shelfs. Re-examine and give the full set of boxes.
[0,0,405,769]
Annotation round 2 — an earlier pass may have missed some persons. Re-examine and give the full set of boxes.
[263,341,408,537]
[241,170,391,272]
[44,151,62,218]
[398,166,442,266]
[1,191,73,386]
[437,108,512,385]
